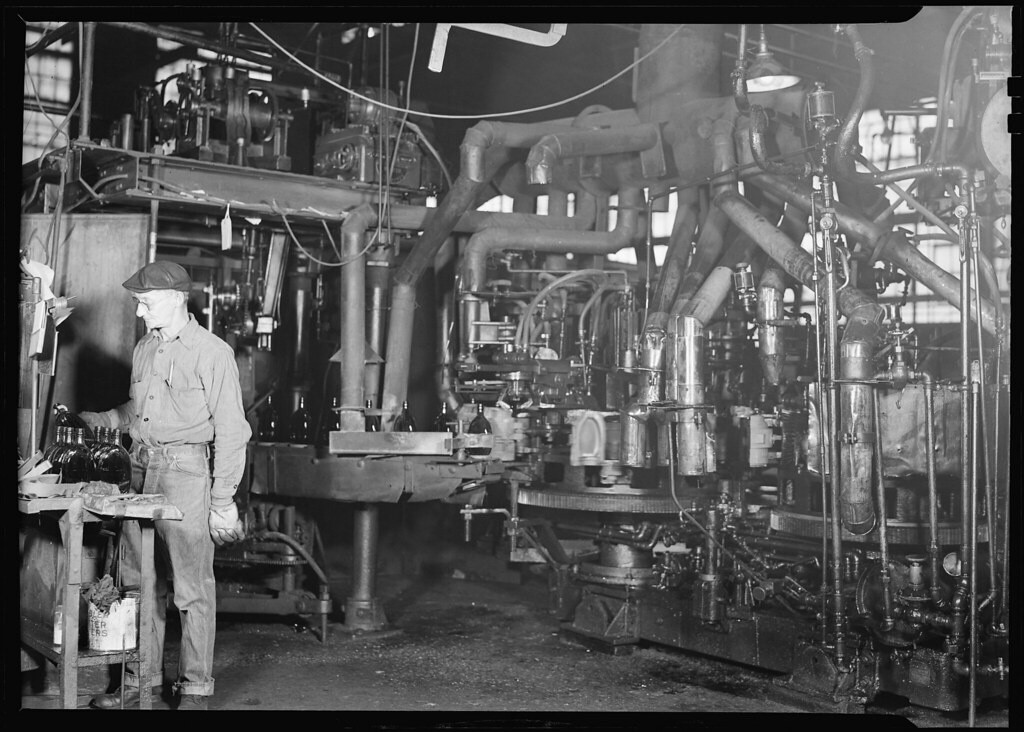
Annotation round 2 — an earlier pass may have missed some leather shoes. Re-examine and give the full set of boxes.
[177,695,208,710]
[93,681,162,709]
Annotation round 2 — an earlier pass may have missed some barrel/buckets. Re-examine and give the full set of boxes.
[87,593,140,651]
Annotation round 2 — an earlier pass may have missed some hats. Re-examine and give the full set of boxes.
[122,261,192,291]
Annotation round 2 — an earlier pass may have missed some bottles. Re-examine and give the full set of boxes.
[42,403,131,494]
[54,602,63,645]
[365,400,380,432]
[469,403,492,454]
[322,397,340,445]
[433,402,457,454]
[394,401,416,433]
[258,395,280,442]
[290,397,311,443]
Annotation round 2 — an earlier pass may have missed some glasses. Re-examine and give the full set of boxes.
[132,291,176,311]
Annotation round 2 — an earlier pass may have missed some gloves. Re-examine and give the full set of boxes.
[208,502,245,546]
[77,411,111,434]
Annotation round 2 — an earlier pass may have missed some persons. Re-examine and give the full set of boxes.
[78,260,252,709]
